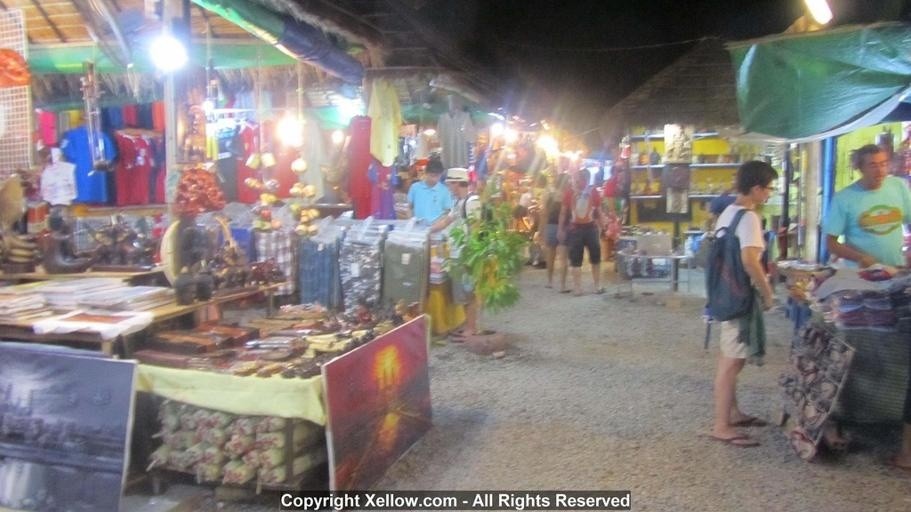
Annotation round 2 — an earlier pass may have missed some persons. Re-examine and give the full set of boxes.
[822,145,911,267]
[532,167,616,296]
[711,161,779,447]
[710,183,769,365]
[430,168,482,340]
[406,160,454,240]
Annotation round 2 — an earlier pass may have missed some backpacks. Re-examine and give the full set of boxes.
[702,209,754,350]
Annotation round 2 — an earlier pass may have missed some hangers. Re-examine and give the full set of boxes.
[212,110,255,131]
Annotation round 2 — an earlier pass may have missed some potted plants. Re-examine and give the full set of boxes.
[448,198,527,353]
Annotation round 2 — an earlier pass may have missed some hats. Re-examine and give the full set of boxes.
[444,169,469,182]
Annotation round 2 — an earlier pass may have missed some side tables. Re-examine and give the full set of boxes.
[2,266,433,509]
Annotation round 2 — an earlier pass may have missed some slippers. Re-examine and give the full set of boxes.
[883,455,911,469]
[771,325,854,462]
[728,417,767,426]
[708,434,760,447]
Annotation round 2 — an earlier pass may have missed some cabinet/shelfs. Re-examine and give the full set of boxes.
[630,128,802,262]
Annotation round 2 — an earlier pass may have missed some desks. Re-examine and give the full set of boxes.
[785,286,911,427]
[616,250,695,304]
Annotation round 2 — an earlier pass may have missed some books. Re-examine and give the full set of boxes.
[0,278,175,322]
